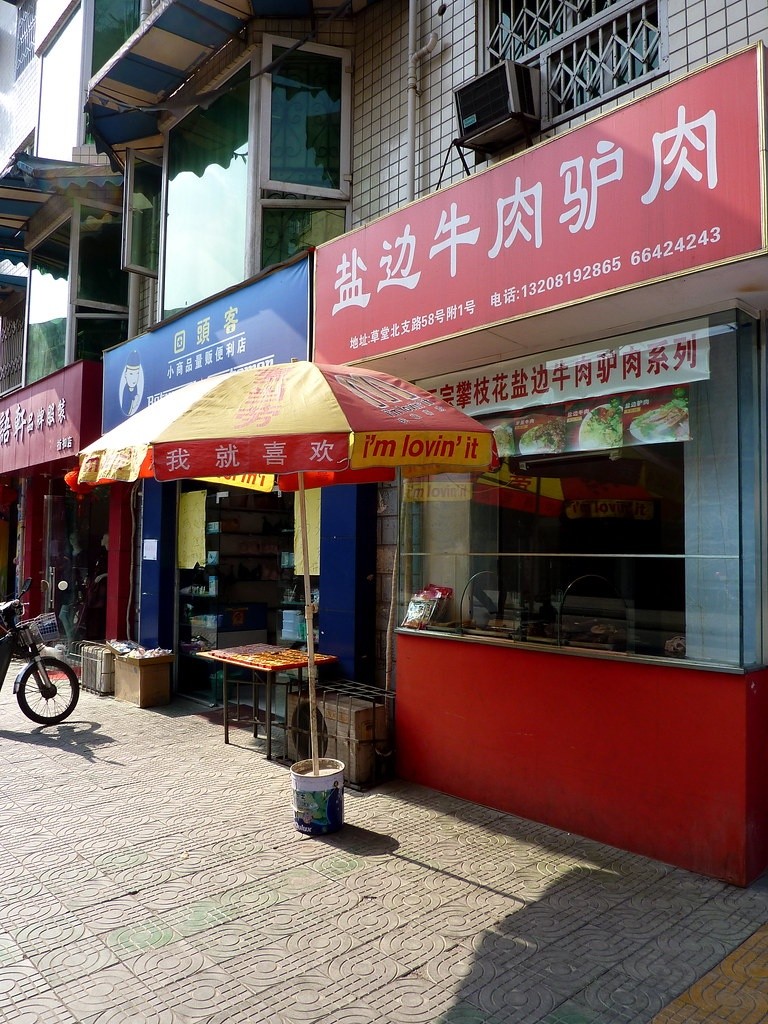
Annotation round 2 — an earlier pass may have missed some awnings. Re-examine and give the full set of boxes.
[0,152,123,282]
[85,2,248,176]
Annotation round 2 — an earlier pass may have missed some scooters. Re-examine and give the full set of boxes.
[0,576,79,724]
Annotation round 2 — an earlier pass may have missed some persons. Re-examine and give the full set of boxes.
[59,530,85,660]
[89,533,108,642]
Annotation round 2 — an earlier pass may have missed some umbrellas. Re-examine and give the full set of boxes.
[78,360,501,775]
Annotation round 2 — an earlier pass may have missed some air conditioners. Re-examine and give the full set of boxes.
[80,645,117,693]
[282,690,384,785]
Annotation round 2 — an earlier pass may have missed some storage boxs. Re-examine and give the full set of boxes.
[272,672,309,723]
[113,658,169,707]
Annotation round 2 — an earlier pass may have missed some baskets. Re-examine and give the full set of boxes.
[15,613,60,647]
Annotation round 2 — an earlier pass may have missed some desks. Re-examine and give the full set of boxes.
[195,642,337,760]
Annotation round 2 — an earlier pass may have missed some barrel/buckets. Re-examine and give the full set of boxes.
[290,757,346,837]
